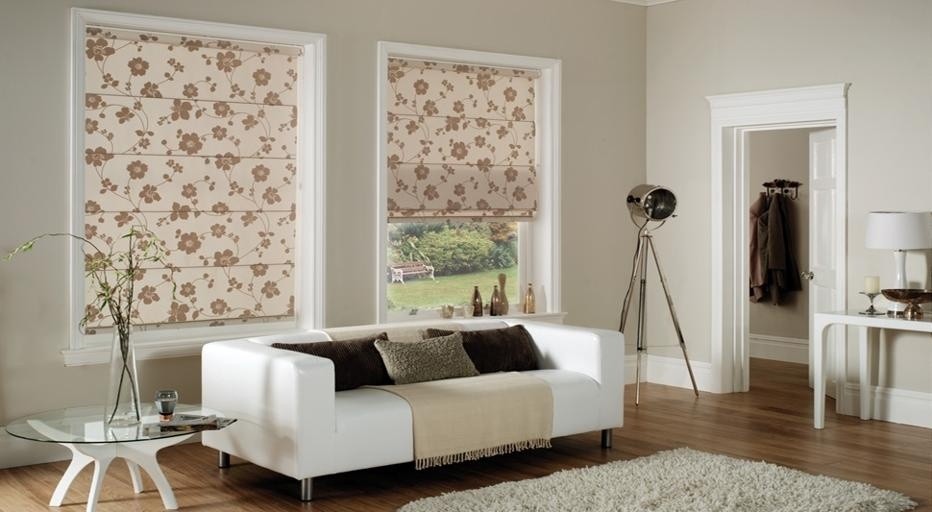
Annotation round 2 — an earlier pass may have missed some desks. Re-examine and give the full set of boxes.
[811,312,932,430]
[4,402,227,512]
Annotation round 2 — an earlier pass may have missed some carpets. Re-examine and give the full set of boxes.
[395,445,919,512]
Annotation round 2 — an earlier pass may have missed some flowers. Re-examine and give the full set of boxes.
[0,225,178,424]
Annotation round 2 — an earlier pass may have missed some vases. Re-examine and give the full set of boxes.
[104,323,142,427]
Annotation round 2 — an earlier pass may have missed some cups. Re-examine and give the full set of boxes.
[154,389,178,421]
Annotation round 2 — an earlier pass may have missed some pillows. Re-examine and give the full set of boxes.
[373,331,480,384]
[425,325,540,372]
[269,332,396,392]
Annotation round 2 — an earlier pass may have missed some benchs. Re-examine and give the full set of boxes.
[387,260,438,284]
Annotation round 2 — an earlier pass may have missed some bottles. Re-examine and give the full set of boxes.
[490,285,502,316]
[471,286,483,316]
[524,283,535,314]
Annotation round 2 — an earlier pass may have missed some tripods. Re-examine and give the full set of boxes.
[618,234,701,407]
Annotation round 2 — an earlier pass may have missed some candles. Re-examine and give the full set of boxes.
[864,274,880,293]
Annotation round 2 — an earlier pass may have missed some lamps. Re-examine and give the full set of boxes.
[865,212,931,315]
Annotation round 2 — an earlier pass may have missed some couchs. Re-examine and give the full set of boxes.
[201,315,625,503]
